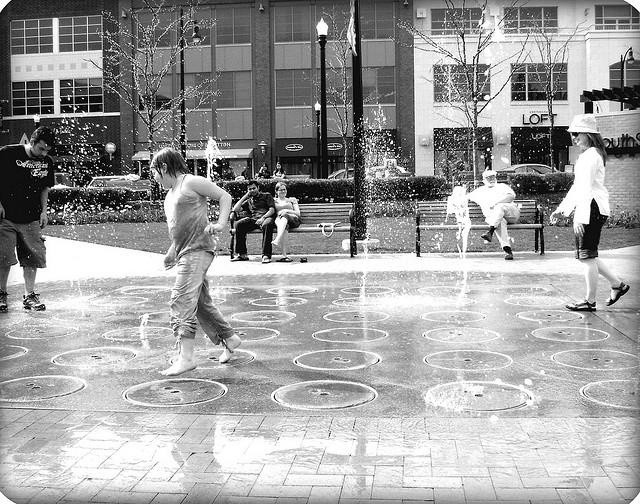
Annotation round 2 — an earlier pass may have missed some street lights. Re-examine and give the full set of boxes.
[32,113,42,129]
[619,44,636,111]
[315,15,332,180]
[314,100,322,179]
[177,5,203,161]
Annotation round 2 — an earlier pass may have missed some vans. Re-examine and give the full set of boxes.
[86,174,151,189]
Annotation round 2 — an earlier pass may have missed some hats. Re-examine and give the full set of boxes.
[567,114,601,133]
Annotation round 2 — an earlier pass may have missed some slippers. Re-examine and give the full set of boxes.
[262,257,271,263]
[300,258,307,262]
[276,257,293,262]
[231,256,249,261]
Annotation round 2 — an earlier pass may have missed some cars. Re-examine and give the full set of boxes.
[367,164,413,179]
[328,166,354,180]
[492,163,575,192]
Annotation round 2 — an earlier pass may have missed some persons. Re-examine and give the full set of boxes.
[150,148,241,376]
[467,171,520,260]
[0,126,55,313]
[212,159,301,263]
[550,113,630,311]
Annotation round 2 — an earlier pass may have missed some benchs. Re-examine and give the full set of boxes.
[415,199,543,255]
[227,201,359,256]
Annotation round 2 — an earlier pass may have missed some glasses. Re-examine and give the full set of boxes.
[572,132,587,137]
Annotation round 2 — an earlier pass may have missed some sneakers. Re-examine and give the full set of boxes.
[23,292,45,311]
[505,253,513,260]
[481,231,492,242]
[0,289,8,313]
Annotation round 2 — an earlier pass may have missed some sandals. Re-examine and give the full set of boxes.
[607,282,630,306]
[566,300,596,311]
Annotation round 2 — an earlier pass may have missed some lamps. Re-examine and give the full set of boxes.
[104,141,117,160]
[258,139,267,156]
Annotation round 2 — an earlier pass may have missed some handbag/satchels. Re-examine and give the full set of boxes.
[319,222,341,237]
